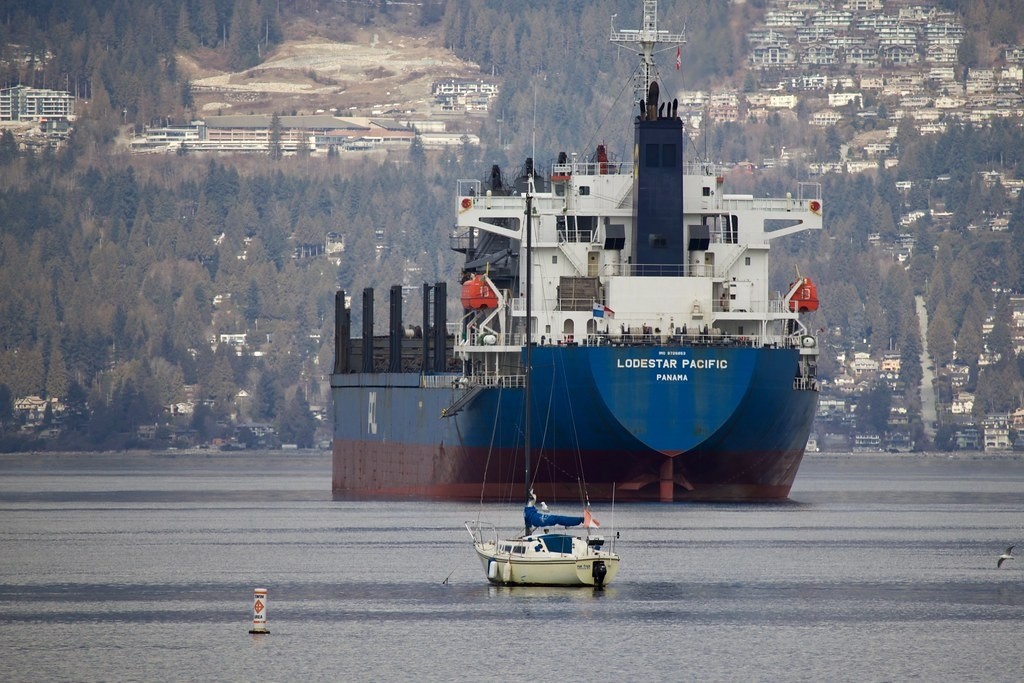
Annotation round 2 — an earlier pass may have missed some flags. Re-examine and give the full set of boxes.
[677,48,682,70]
[593,303,616,319]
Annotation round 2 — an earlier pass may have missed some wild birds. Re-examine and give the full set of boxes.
[996,545,1016,568]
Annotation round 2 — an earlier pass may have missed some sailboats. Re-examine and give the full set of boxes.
[465,166,623,591]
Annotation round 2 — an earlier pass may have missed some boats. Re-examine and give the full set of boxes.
[461,274,499,311]
[324,1,828,504]
[789,274,821,313]
